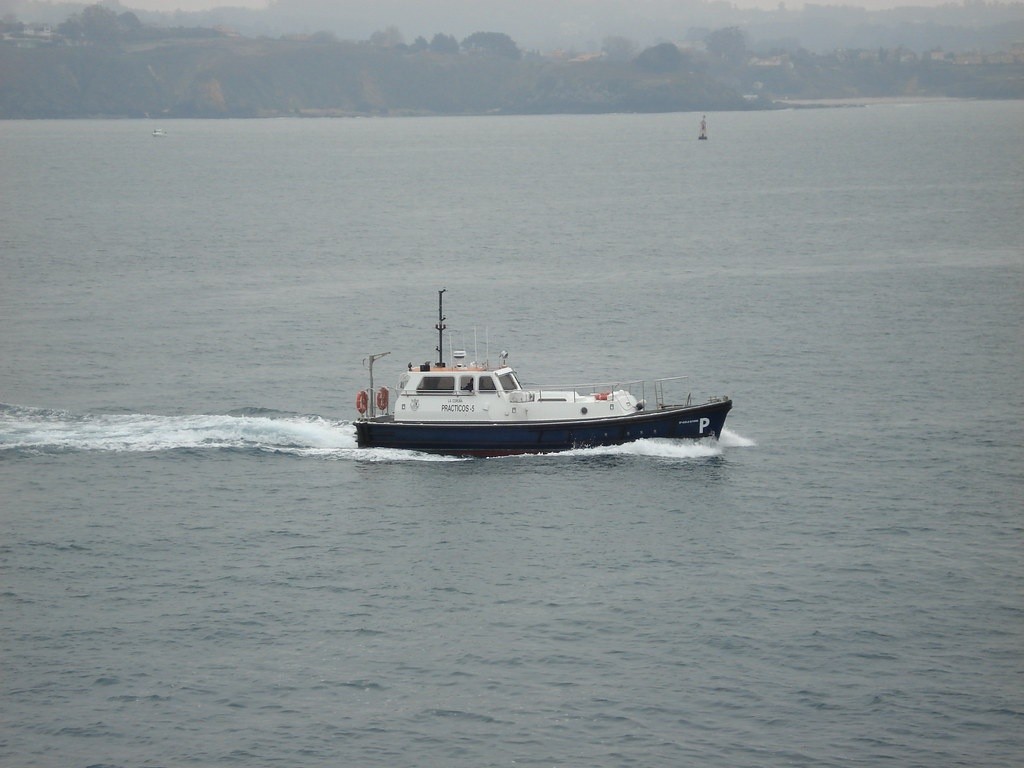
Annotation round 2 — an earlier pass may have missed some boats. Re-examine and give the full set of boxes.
[351,286,735,460]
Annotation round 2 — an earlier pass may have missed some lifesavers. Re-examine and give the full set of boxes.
[595,391,611,400]
[376,387,390,411]
[356,389,369,415]
